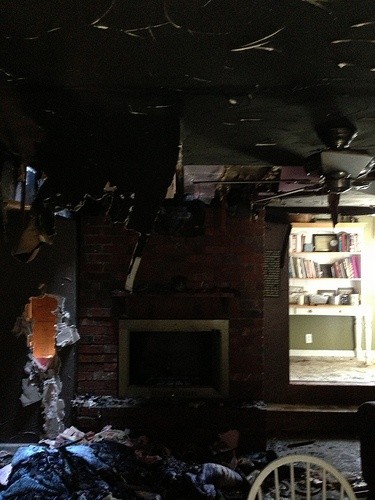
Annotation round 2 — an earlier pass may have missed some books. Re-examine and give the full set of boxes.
[290,232,363,279]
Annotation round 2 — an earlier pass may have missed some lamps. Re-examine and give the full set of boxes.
[314,119,372,194]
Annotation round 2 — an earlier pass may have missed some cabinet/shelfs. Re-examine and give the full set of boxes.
[289,227,371,357]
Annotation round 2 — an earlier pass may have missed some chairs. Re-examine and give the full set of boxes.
[243,401,375,500]
[249,456,357,500]
